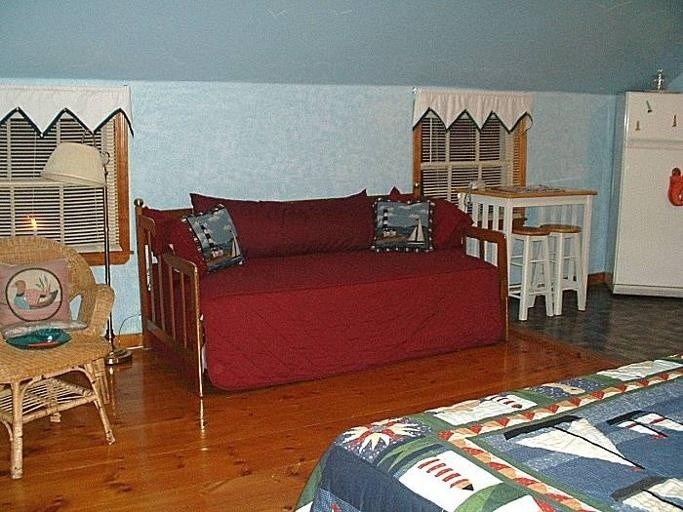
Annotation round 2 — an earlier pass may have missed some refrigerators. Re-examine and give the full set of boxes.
[604,90,683,302]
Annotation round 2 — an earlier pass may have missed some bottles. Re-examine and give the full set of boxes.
[653,69,663,90]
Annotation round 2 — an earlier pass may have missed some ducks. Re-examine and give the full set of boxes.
[10,280,58,309]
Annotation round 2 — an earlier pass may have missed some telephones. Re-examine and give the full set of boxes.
[468,180,485,189]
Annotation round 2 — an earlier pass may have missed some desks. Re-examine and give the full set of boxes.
[468,186,598,306]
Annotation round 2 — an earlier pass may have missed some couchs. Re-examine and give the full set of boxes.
[134,193,510,398]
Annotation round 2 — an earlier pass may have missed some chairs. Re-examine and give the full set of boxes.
[0,234,117,480]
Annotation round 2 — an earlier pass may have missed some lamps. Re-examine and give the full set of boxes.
[39,127,133,366]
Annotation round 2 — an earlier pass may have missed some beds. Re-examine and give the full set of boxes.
[326,351,683,511]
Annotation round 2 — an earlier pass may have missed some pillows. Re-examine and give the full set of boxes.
[370,197,435,254]
[169,204,245,273]
[436,196,468,253]
[190,186,376,256]
[0,259,71,330]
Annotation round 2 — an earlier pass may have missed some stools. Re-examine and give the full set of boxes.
[512,223,587,321]
